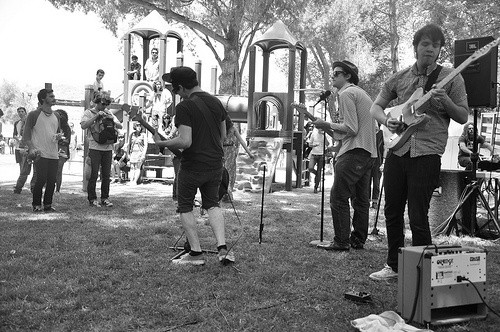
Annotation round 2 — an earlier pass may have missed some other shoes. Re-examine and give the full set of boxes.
[218,248,236,262]
[101,198,113,207]
[171,253,205,265]
[89,200,102,208]
[33,205,56,213]
[372,203,378,209]
[317,241,350,251]
[349,237,364,249]
[369,263,398,281]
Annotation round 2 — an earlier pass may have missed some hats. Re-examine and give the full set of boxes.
[332,60,359,85]
[161,66,197,85]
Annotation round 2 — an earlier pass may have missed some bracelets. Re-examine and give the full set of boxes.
[385,117,389,127]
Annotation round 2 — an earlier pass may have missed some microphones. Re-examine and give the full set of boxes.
[313,90,331,107]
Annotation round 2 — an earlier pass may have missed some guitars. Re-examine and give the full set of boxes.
[291,103,341,151]
[121,104,229,201]
[383,38,500,151]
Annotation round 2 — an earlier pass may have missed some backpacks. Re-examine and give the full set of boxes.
[88,108,118,145]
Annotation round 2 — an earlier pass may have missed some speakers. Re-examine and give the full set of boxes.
[398,246,489,326]
[427,170,472,234]
[453,36,498,107]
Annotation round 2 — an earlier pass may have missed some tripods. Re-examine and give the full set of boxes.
[169,237,218,263]
[433,107,500,237]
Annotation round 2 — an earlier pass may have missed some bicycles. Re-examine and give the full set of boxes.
[477,154,500,211]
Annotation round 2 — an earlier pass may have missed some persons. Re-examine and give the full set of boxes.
[67,123,77,173]
[89,47,172,186]
[458,121,498,181]
[303,121,329,193]
[370,22,469,280]
[310,59,378,252]
[80,90,122,207]
[0,109,4,139]
[152,67,235,264]
[13,88,71,212]
[167,95,255,203]
[369,122,384,210]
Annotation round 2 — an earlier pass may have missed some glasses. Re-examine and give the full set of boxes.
[333,71,347,77]
[163,118,170,119]
[172,86,181,94]
[100,101,109,106]
